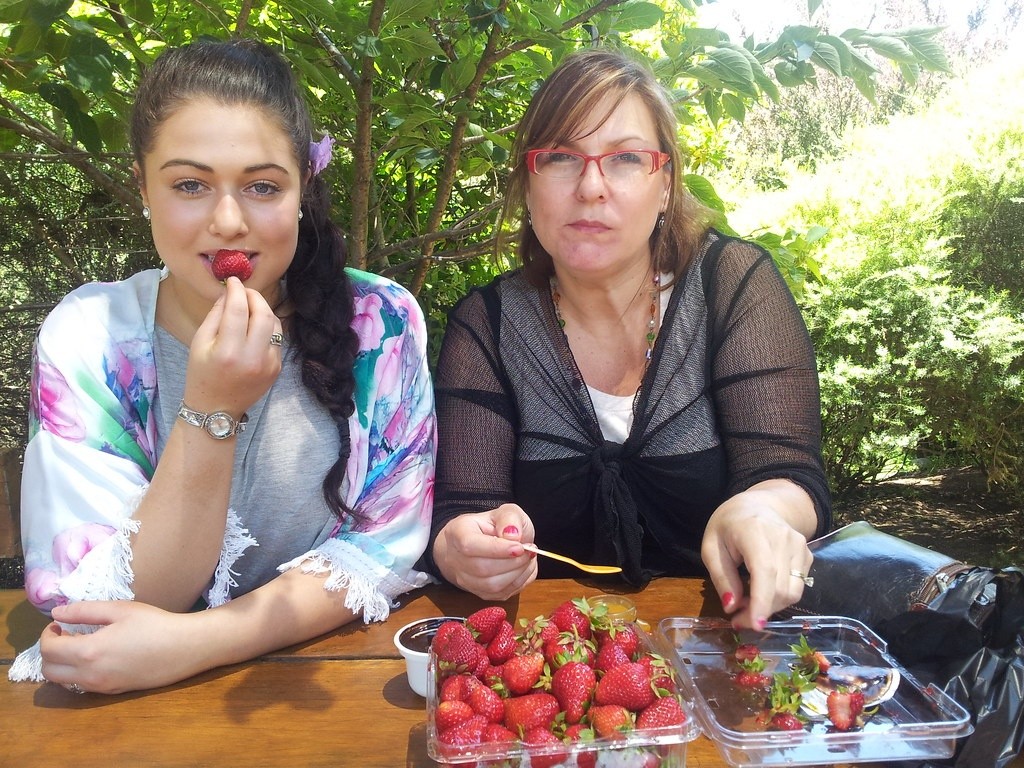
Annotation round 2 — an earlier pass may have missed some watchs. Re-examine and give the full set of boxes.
[177,400,249,440]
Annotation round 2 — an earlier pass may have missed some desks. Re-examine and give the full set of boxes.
[0,575,857,768]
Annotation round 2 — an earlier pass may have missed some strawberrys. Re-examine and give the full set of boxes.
[733,645,863,730]
[431,601,687,768]
[212,249,252,282]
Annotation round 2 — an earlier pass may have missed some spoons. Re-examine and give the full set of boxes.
[503,539,622,578]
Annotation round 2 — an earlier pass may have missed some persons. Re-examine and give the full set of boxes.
[414,47,835,641]
[7,37,439,697]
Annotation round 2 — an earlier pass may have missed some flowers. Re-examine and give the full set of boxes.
[308,133,334,180]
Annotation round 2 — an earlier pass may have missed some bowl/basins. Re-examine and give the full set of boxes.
[656,614,975,766]
[423,629,703,767]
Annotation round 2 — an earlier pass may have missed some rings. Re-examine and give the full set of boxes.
[790,569,814,587]
[270,332,283,346]
[70,683,85,695]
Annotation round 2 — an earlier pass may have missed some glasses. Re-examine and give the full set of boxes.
[525,149,670,180]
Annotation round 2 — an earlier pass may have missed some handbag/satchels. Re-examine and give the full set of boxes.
[744,518,1024,768]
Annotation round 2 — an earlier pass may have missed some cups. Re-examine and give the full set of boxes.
[393,616,475,698]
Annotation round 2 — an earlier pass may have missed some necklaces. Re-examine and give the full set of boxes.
[551,271,659,380]
